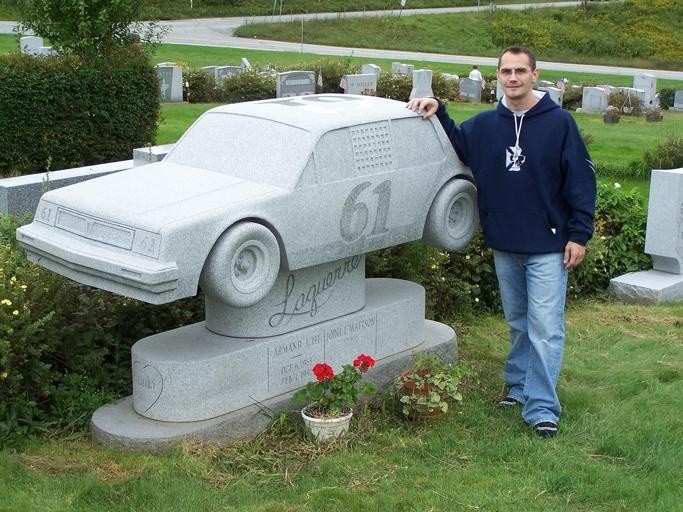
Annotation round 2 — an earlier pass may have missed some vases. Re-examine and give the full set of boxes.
[301,402,353,446]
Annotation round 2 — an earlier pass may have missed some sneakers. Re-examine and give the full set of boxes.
[499,397,517,405]
[535,422,558,434]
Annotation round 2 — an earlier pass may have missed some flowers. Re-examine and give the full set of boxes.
[292,353,377,414]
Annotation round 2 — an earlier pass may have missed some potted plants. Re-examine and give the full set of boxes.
[382,349,482,420]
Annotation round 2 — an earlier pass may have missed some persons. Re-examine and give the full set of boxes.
[403,46,597,439]
[468,65,482,81]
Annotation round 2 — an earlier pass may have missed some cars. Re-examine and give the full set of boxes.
[13,91,488,309]
